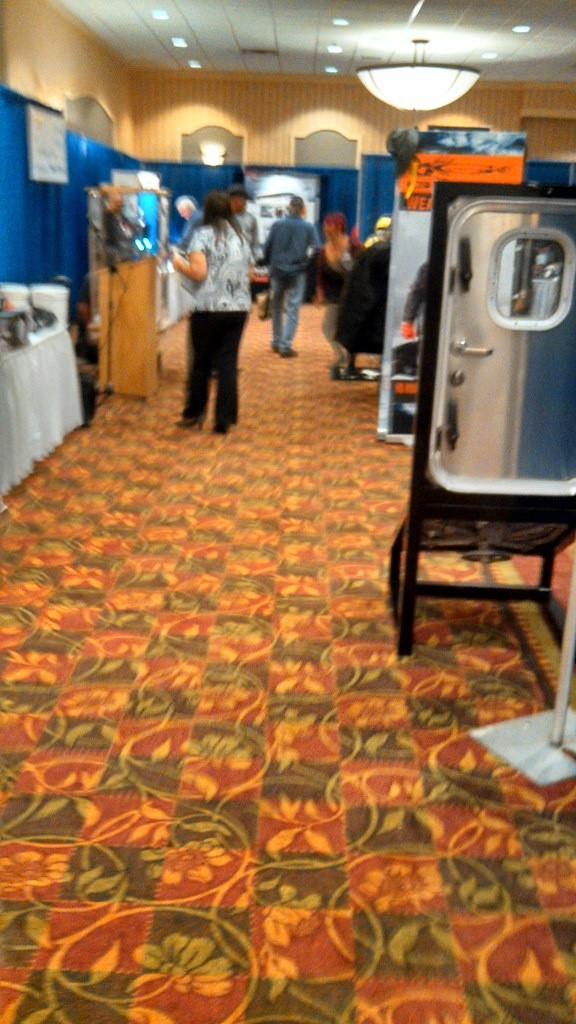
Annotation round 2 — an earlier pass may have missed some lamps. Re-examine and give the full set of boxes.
[355,34,483,116]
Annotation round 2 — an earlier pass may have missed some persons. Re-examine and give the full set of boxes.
[364,214,392,251]
[170,195,219,379]
[317,212,368,306]
[229,194,257,282]
[263,197,322,357]
[170,192,255,436]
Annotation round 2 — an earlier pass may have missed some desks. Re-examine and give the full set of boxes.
[0,316,87,516]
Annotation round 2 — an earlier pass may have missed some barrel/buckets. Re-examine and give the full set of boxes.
[0,283,70,326]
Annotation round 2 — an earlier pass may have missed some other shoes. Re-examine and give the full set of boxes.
[280,347,298,358]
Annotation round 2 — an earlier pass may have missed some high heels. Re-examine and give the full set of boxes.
[174,415,203,430]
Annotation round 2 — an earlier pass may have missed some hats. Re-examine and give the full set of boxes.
[228,185,253,201]
